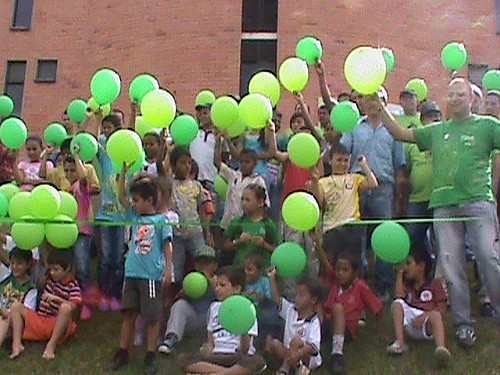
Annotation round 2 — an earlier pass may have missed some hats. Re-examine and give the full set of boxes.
[399,89,418,100]
[195,103,212,111]
[193,246,215,259]
[421,101,440,114]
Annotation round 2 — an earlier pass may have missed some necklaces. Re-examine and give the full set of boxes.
[331,170,347,193]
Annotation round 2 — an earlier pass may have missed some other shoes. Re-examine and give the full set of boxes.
[331,353,345,374]
[158,340,175,354]
[133,332,142,346]
[455,323,476,348]
[386,341,407,352]
[110,297,120,311]
[435,347,450,367]
[108,349,128,370]
[141,358,158,375]
[296,365,310,375]
[358,317,365,327]
[376,290,391,304]
[80,303,91,320]
[97,297,109,312]
[481,302,495,317]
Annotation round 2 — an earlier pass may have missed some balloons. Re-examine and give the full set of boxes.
[482,69,500,91]
[329,99,360,133]
[441,41,468,71]
[405,77,428,101]
[270,241,307,277]
[343,45,394,96]
[371,221,410,263]
[0,35,323,250]
[219,294,256,335]
[183,271,208,298]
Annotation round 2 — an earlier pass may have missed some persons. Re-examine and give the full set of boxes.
[0,58,500,375]
